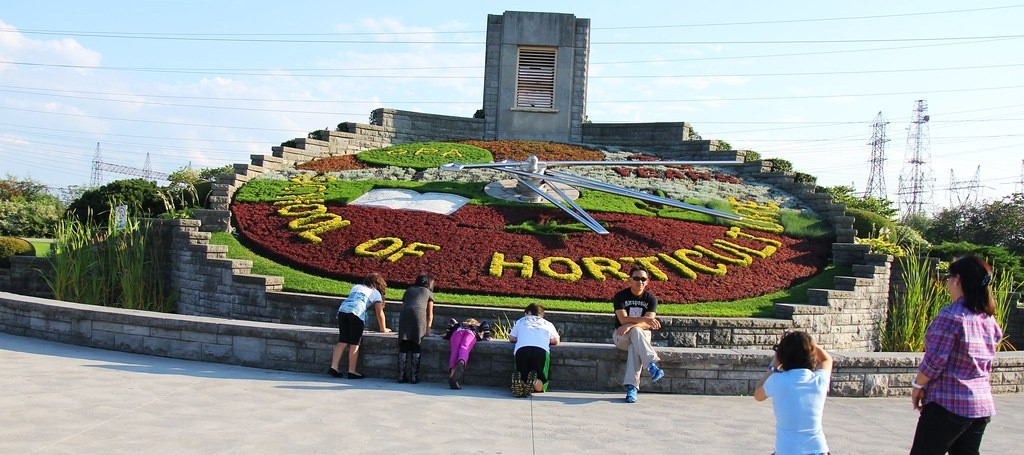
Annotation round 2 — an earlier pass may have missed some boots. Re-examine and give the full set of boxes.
[398,352,409,383]
[410,353,421,384]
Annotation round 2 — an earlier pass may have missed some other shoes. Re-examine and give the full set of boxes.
[511,371,523,397]
[448,376,461,390]
[328,368,343,377]
[348,372,364,379]
[452,363,464,382]
[522,371,537,397]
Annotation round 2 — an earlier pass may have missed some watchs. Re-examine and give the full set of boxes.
[912,378,926,389]
[768,365,778,372]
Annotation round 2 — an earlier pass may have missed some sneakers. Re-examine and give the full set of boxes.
[625,385,638,402]
[646,363,664,381]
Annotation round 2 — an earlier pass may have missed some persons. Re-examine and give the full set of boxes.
[440,318,494,389]
[613,267,664,402]
[398,274,434,384]
[909,256,1003,455]
[509,303,560,398]
[328,273,391,379]
[754,330,833,455]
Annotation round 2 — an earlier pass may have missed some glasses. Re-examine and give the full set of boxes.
[944,273,957,281]
[632,277,647,282]
